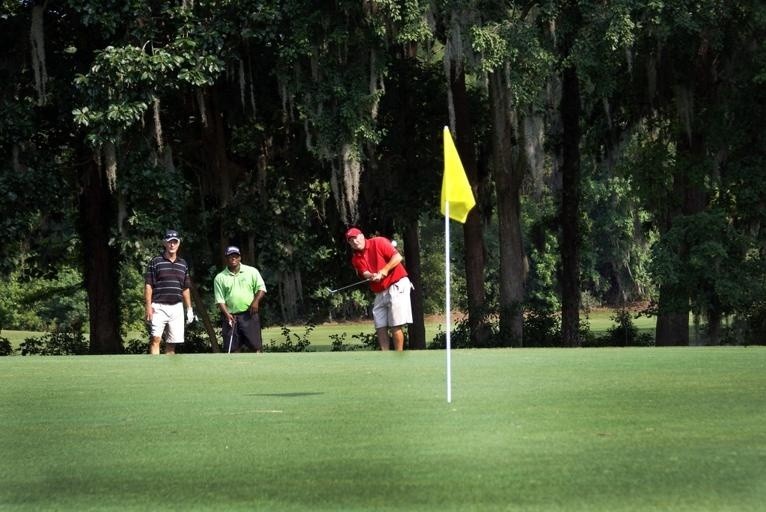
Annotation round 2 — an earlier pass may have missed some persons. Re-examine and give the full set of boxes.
[345,227,414,350]
[213,246,268,352]
[144,229,195,354]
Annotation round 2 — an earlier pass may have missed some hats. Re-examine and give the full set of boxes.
[225,246,240,256]
[346,228,361,243]
[164,230,179,242]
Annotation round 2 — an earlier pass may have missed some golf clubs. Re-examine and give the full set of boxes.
[326,280,369,295]
[227,315,236,352]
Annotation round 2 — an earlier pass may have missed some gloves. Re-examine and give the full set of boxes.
[186,307,195,324]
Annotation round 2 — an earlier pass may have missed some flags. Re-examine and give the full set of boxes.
[440,125,477,224]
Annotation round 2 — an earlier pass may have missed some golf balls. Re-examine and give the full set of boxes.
[392,241,397,247]
